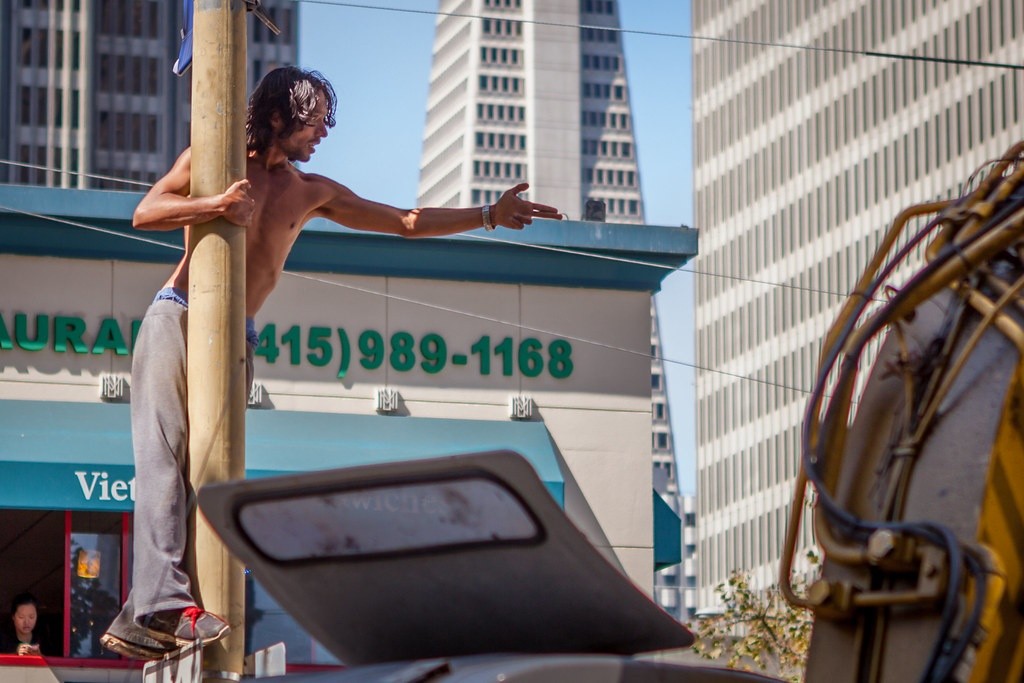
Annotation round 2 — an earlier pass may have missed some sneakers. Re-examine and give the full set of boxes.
[100,634,162,659]
[142,606,231,647]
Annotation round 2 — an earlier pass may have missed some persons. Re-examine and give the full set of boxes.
[0,593,63,657]
[101,67,562,659]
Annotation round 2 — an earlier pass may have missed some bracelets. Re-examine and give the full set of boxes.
[482,205,495,231]
